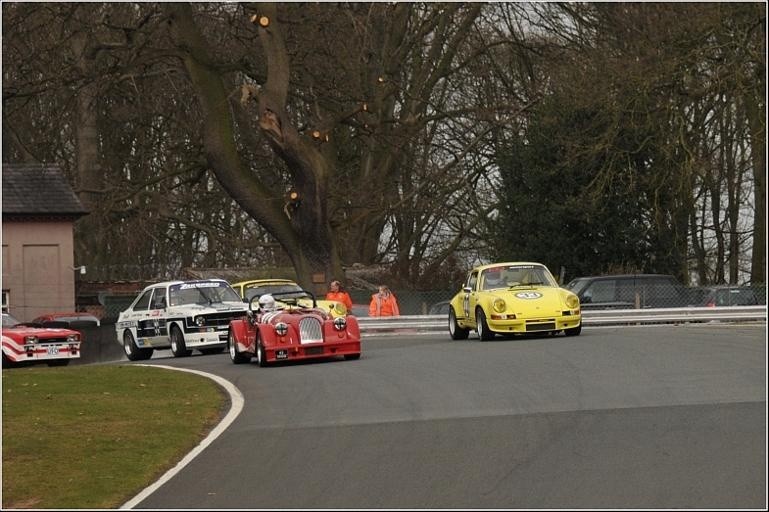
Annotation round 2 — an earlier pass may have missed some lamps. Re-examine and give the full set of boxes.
[67,264,87,275]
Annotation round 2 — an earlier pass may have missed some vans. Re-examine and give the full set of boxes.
[559,273,689,311]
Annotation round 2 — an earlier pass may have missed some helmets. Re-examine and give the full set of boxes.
[257,294,276,314]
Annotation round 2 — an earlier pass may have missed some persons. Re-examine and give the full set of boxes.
[325,281,352,315]
[254,293,274,322]
[368,286,400,316]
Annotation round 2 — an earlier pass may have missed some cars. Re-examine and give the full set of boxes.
[214,276,349,324]
[112,277,254,362]
[25,310,102,330]
[426,299,451,315]
[2,311,86,368]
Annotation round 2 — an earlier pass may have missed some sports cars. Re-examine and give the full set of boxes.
[223,287,363,369]
[447,260,582,343]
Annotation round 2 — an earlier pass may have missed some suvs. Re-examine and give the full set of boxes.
[683,282,761,323]
